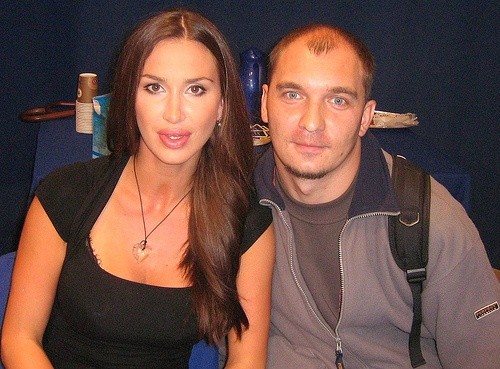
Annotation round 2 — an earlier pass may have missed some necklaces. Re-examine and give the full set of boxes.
[132,151,194,261]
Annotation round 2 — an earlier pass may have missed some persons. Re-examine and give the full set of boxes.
[246,23,500,369]
[0,8,275,369]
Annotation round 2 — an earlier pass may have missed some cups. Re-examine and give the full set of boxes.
[75,72,98,134]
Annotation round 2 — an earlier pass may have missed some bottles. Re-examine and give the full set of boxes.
[239,48,266,117]
